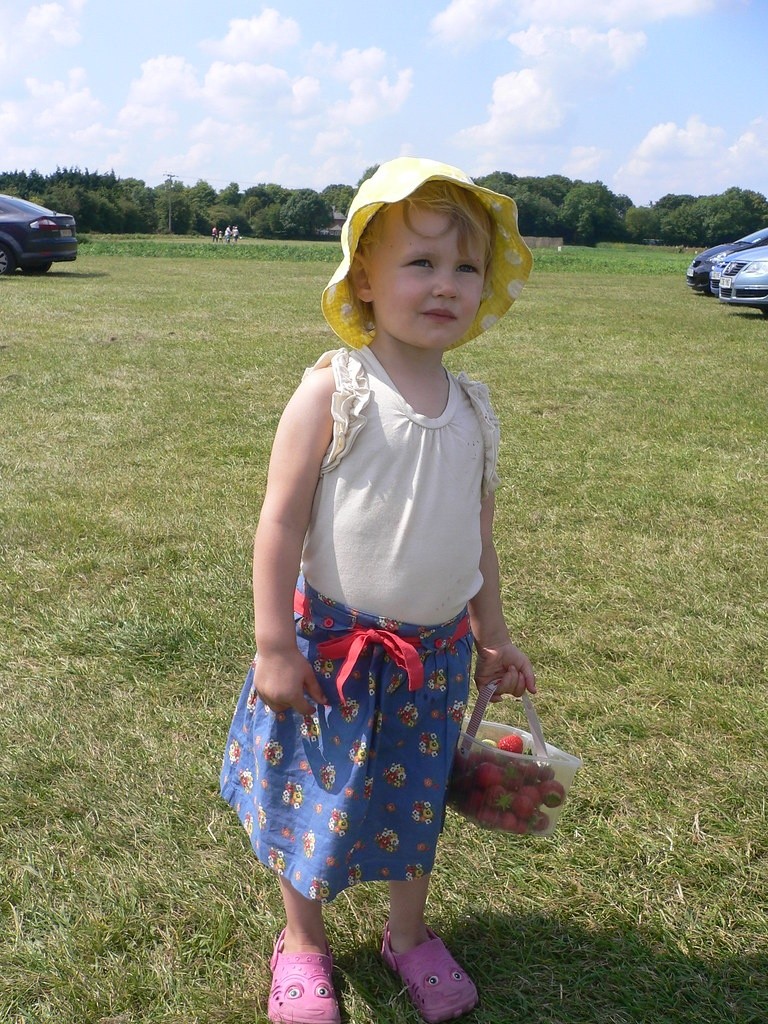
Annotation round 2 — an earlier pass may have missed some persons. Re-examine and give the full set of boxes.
[247,165,537,1023]
[211,224,242,247]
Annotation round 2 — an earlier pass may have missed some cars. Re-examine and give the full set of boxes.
[686,227,768,316]
[0,194,78,275]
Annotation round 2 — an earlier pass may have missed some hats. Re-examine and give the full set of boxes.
[312,162,534,357]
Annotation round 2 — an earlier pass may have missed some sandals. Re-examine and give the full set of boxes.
[378,919,479,1022]
[266,926,342,1024]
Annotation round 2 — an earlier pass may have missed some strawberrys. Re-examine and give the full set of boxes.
[447,734,565,833]
